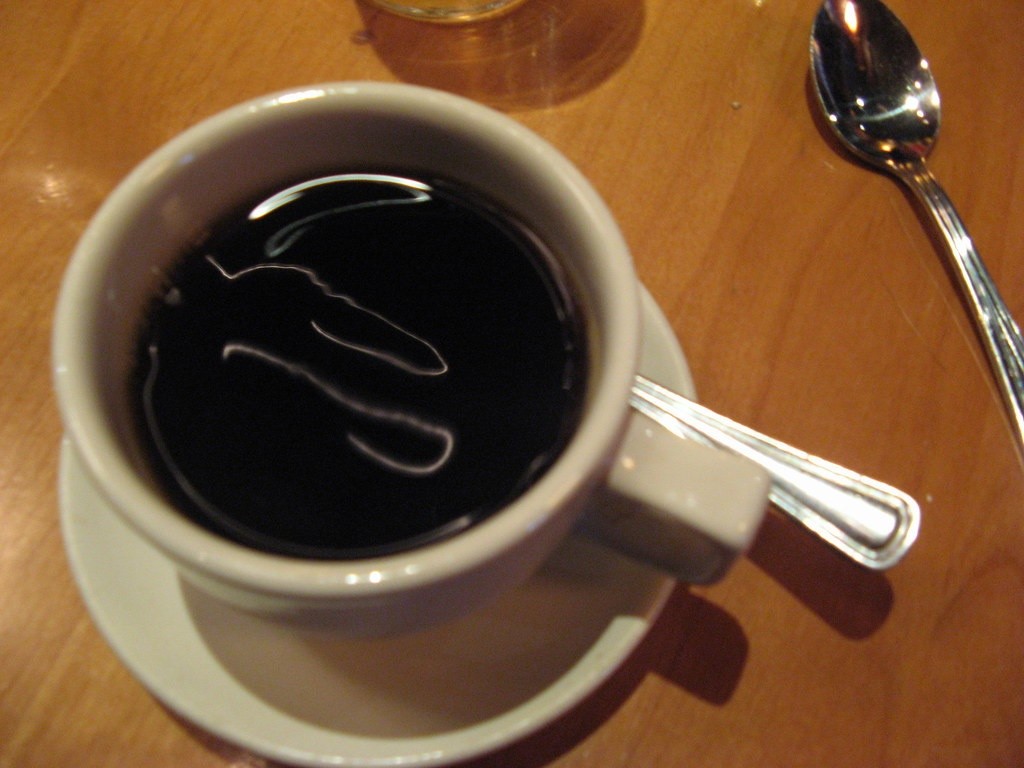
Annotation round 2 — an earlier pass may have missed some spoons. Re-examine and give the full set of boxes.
[809,0,1024,445]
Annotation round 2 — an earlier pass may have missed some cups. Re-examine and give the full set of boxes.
[360,0,527,25]
[50,79,772,616]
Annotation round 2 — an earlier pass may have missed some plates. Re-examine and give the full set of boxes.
[59,272,696,768]
